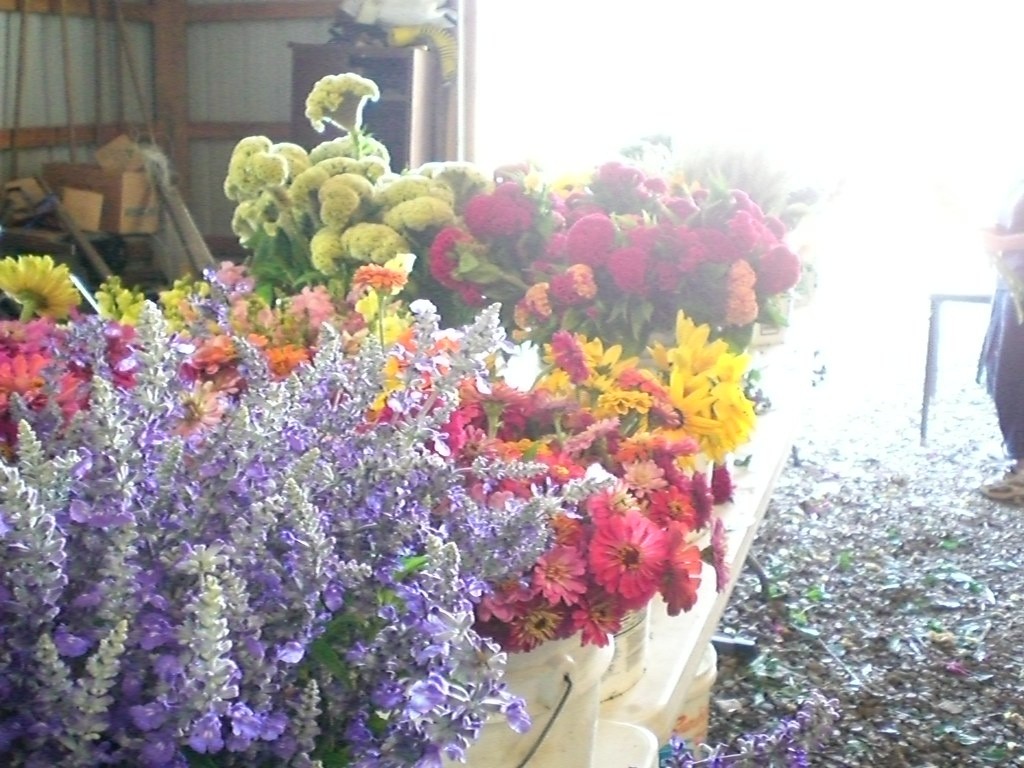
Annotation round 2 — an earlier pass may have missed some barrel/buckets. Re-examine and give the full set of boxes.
[600,602,647,701]
[439,628,616,768]
[673,643,718,747]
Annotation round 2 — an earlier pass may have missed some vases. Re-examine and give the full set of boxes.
[445,599,654,767]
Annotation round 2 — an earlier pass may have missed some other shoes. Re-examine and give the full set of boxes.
[979,466,1024,499]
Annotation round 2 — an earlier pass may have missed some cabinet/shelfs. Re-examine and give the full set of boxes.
[289,40,438,175]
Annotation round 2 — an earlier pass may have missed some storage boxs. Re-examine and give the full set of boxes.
[41,159,163,234]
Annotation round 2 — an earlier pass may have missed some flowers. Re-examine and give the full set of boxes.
[0,75,805,768]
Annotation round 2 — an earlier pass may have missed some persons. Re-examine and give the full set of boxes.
[975,197,1024,502]
